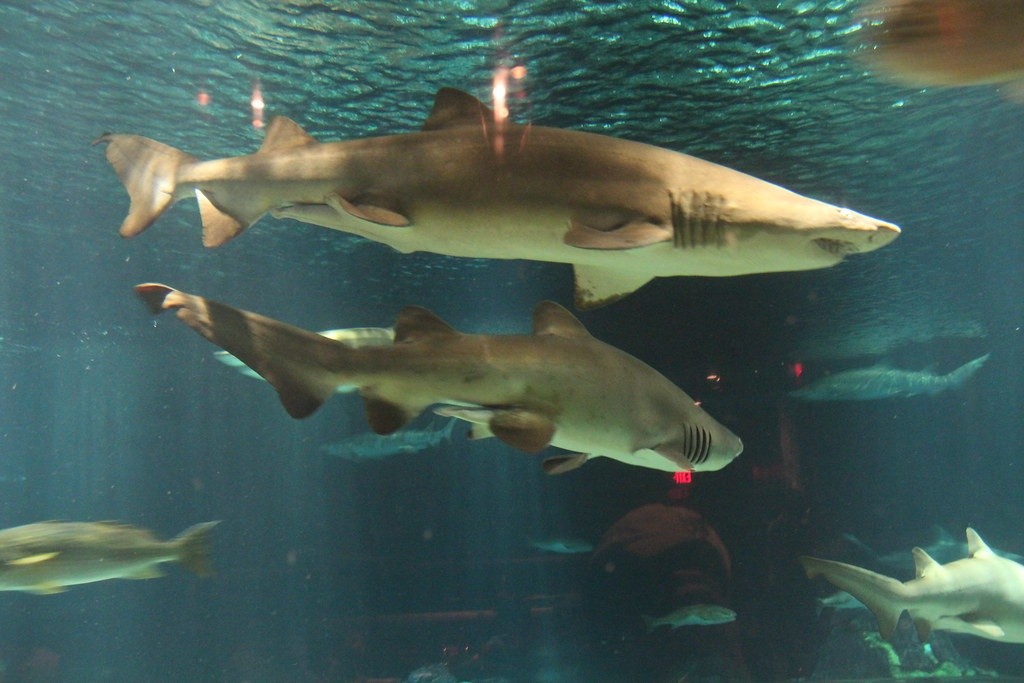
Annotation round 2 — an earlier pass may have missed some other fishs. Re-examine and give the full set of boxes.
[0,518,221,597]
[524,501,737,632]
